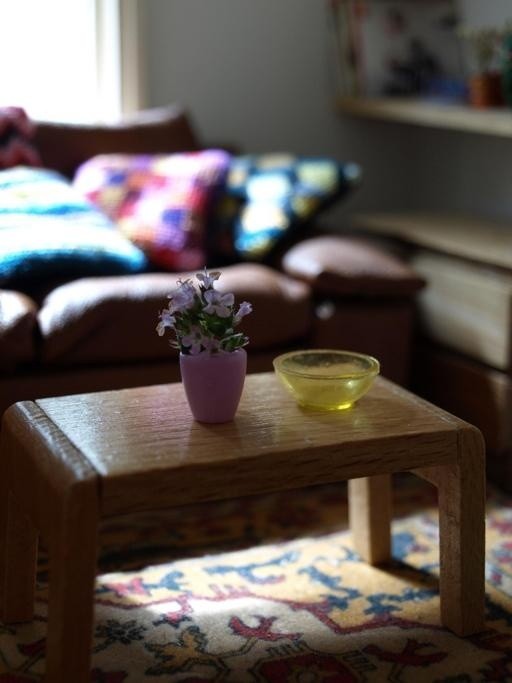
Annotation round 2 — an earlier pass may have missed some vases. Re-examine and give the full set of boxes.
[180,350,248,424]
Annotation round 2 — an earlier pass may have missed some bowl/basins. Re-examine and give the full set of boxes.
[273,347,382,413]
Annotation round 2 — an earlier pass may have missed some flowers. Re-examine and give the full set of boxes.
[156,265,253,354]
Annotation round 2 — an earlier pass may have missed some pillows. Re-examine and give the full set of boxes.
[71,148,230,270]
[211,149,367,261]
[0,165,147,285]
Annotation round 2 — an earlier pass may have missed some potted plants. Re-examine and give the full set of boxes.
[456,27,504,107]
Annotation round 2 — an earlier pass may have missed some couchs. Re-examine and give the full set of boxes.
[1,106,425,409]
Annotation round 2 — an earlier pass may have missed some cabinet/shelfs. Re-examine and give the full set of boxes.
[333,91,512,491]
